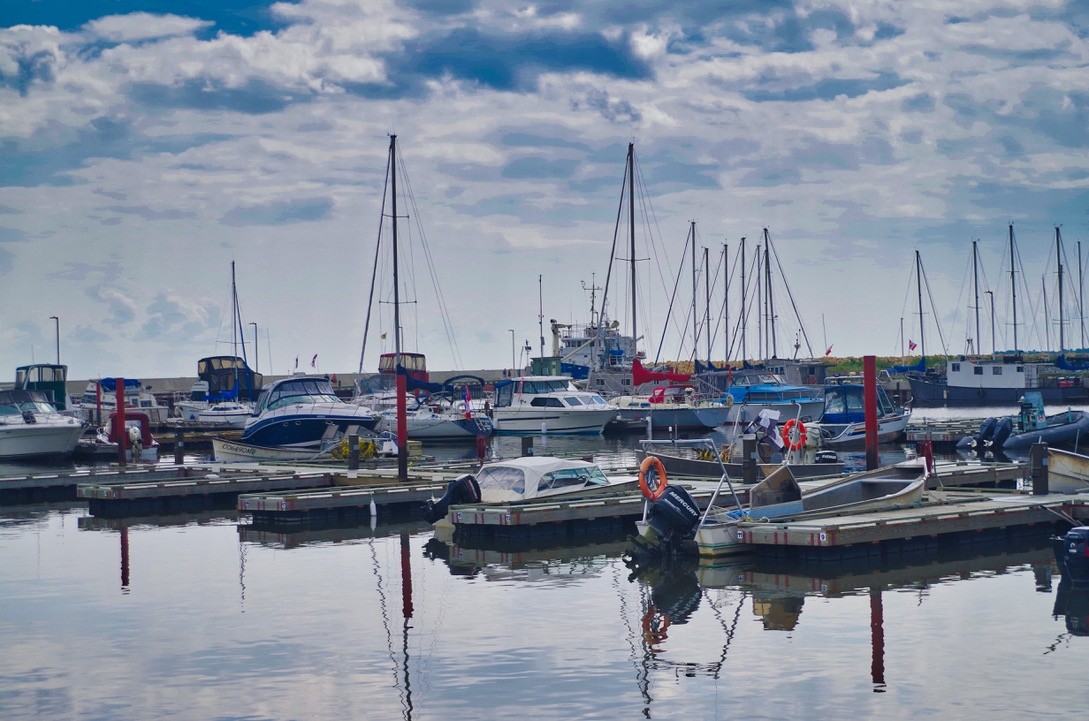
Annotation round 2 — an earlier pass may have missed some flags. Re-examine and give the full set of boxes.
[909,340,917,352]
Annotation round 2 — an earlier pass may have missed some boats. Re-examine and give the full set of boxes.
[605,387,728,430]
[695,455,932,547]
[75,408,161,463]
[240,375,381,454]
[433,456,644,547]
[0,385,86,463]
[802,381,911,445]
[350,377,487,443]
[477,375,617,435]
[1051,523,1089,584]
[906,359,1088,406]
[952,408,1089,494]
[721,381,824,422]
[69,390,410,428]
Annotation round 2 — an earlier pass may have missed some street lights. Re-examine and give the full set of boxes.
[249,321,258,378]
[510,328,515,375]
[51,315,62,367]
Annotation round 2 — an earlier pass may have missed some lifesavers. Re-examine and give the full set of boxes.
[639,455,668,500]
[727,394,735,406]
[782,419,808,450]
